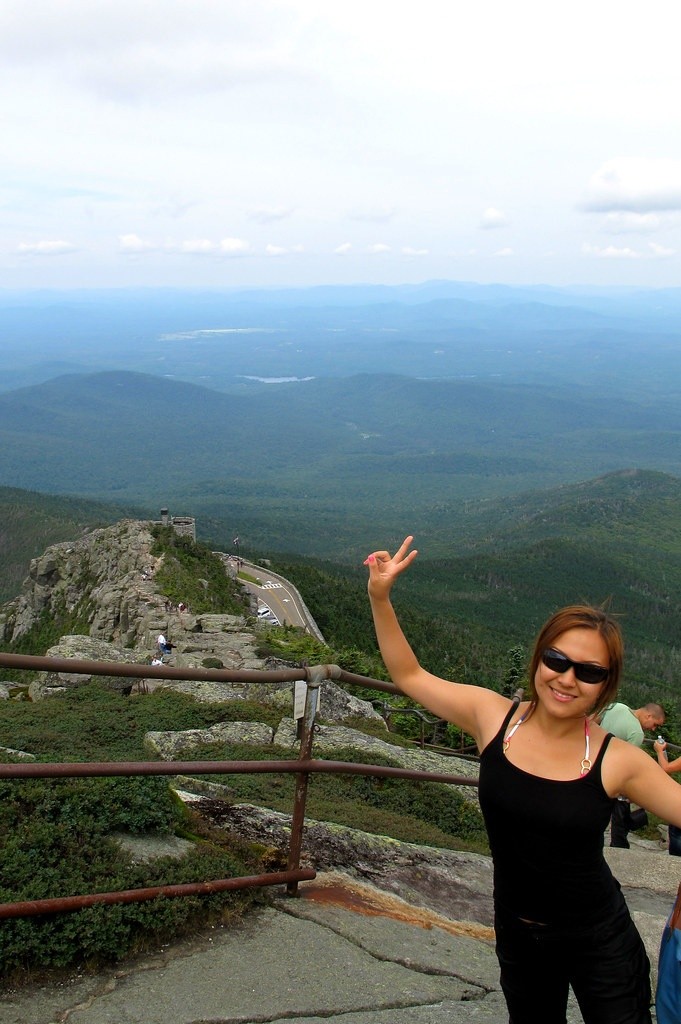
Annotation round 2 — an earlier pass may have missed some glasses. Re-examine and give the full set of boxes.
[541,648,608,684]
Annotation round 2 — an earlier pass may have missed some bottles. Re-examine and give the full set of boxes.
[657,735,668,760]
[617,795,630,802]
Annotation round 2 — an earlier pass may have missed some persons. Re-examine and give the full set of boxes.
[158,632,167,654]
[141,573,147,581]
[363,535,681,1024]
[237,557,243,567]
[588,702,681,856]
[165,599,186,614]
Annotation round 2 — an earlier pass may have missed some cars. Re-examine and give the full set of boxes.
[270,619,279,626]
[258,608,270,618]
